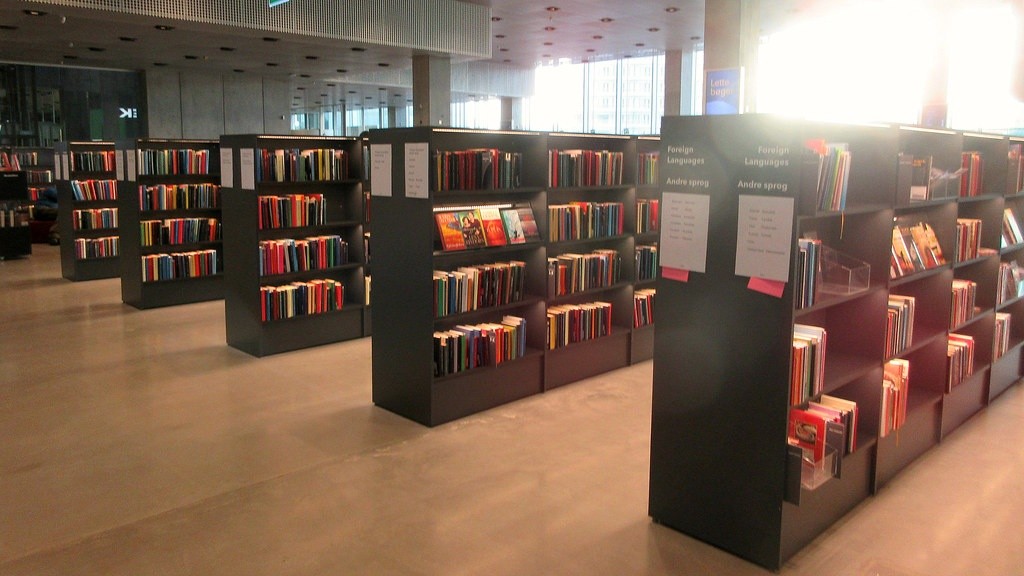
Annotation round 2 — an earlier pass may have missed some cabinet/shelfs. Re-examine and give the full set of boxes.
[368,126,660,427]
[0,146,57,259]
[648,115,1024,572]
[114,138,224,310]
[219,131,371,358]
[54,141,120,282]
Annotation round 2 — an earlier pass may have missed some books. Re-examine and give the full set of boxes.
[138,149,221,282]
[0,152,53,228]
[788,138,1024,483]
[256,148,371,321]
[434,147,661,376]
[71,151,120,259]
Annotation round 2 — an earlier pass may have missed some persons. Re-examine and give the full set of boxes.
[462,217,479,248]
[468,212,485,246]
